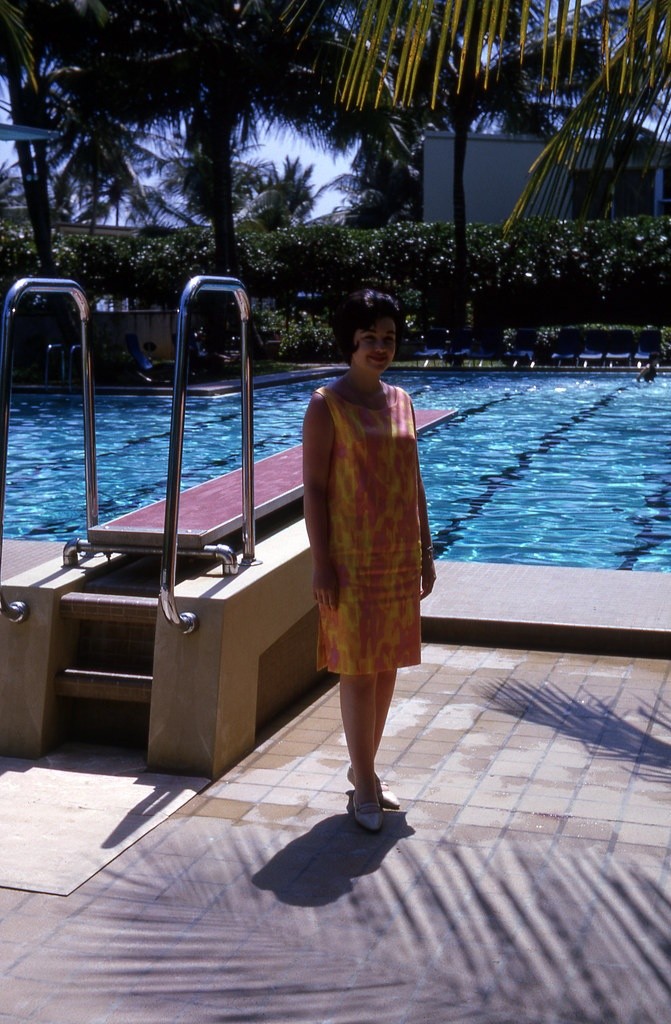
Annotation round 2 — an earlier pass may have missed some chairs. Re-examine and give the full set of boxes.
[503,328,537,365]
[549,328,582,366]
[605,329,633,366]
[413,328,444,367]
[442,328,473,368]
[125,333,171,384]
[578,330,607,366]
[634,330,662,367]
[171,333,225,380]
[470,334,501,367]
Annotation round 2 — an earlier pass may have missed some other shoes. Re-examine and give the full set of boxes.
[347,766,402,808]
[353,793,384,831]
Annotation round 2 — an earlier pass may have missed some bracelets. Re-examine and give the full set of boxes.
[421,545,433,562]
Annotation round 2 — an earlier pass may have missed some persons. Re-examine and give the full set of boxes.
[299,288,437,832]
[637,351,661,384]
